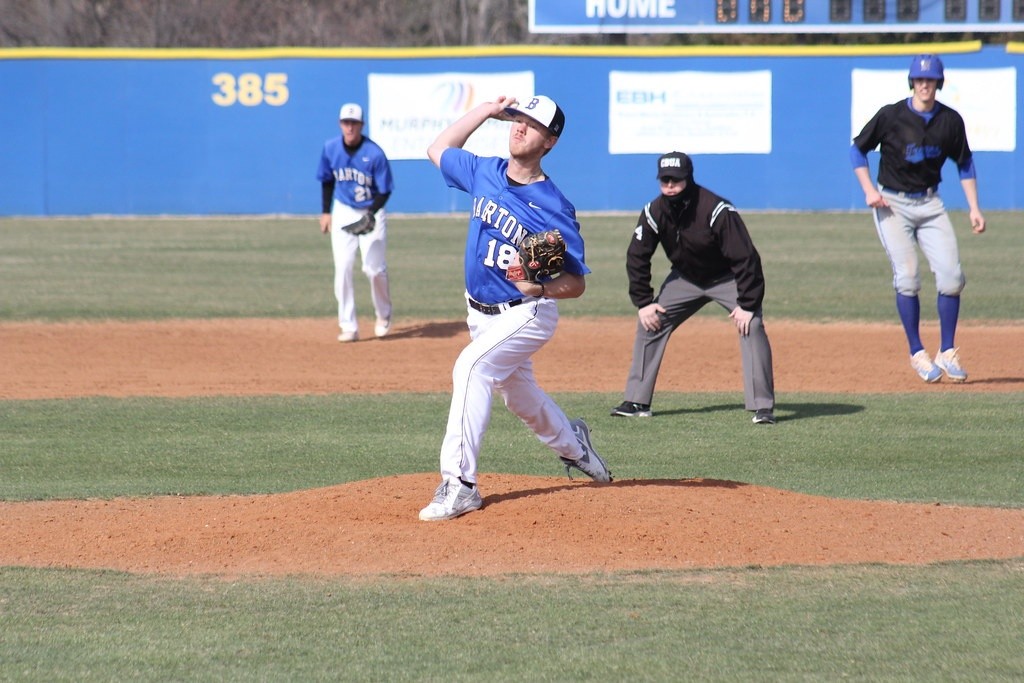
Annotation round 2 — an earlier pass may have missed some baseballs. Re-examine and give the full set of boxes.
[503,98,519,109]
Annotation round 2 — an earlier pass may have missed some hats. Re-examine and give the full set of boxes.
[504,95,566,139]
[340,103,363,122]
[656,151,694,179]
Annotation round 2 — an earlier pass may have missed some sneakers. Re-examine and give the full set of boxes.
[932,347,967,382]
[611,399,652,417]
[374,310,394,337]
[910,348,943,383]
[418,475,482,522]
[337,331,359,343]
[560,418,614,486]
[751,407,774,424]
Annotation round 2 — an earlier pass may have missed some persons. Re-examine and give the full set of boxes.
[849,52,986,383]
[418,93,614,522]
[610,151,775,422]
[315,101,394,343]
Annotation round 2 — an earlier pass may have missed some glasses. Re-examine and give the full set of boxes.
[660,178,681,183]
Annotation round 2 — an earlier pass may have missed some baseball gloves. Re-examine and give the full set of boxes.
[505,226,566,283]
[341,213,376,237]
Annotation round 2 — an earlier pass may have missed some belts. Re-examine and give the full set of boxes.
[883,187,938,200]
[465,298,522,316]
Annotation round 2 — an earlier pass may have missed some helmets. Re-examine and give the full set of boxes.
[908,54,944,91]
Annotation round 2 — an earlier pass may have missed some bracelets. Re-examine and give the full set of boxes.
[533,282,545,299]
